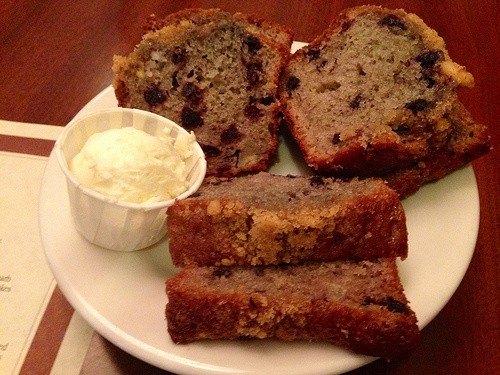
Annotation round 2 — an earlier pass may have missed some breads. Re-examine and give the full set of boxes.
[113,4,494,361]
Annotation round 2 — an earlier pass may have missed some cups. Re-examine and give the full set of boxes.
[55,107,207,253]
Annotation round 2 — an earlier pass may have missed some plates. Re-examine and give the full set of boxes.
[38,37,479,375]
